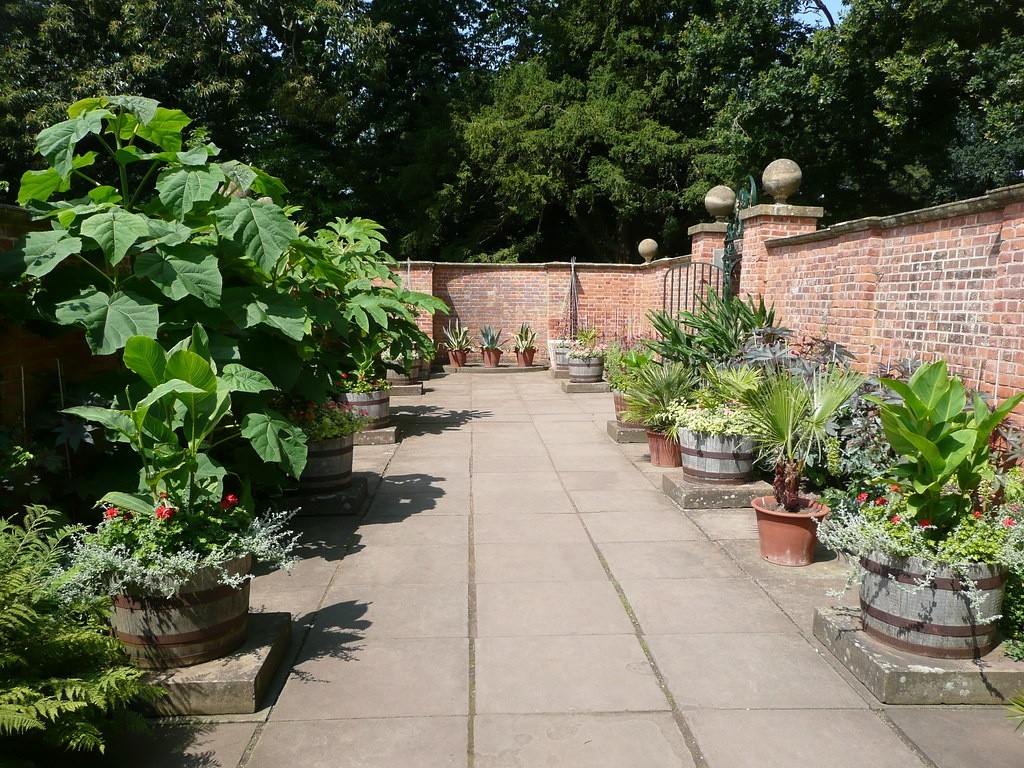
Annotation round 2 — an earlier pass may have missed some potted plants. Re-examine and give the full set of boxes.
[53,316,314,670]
[858,358,1024,660]
[610,373,658,428]
[568,326,607,382]
[507,321,539,367]
[475,325,511,368]
[664,362,765,484]
[438,318,475,367]
[709,354,867,568]
[617,361,702,467]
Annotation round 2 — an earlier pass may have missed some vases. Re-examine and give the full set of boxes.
[297,433,353,494]
[386,359,421,386]
[341,390,391,430]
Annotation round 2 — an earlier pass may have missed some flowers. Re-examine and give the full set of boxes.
[336,373,394,400]
[809,485,1024,661]
[45,487,304,603]
[270,394,374,442]
[381,346,420,364]
[565,340,608,359]
[652,396,770,437]
